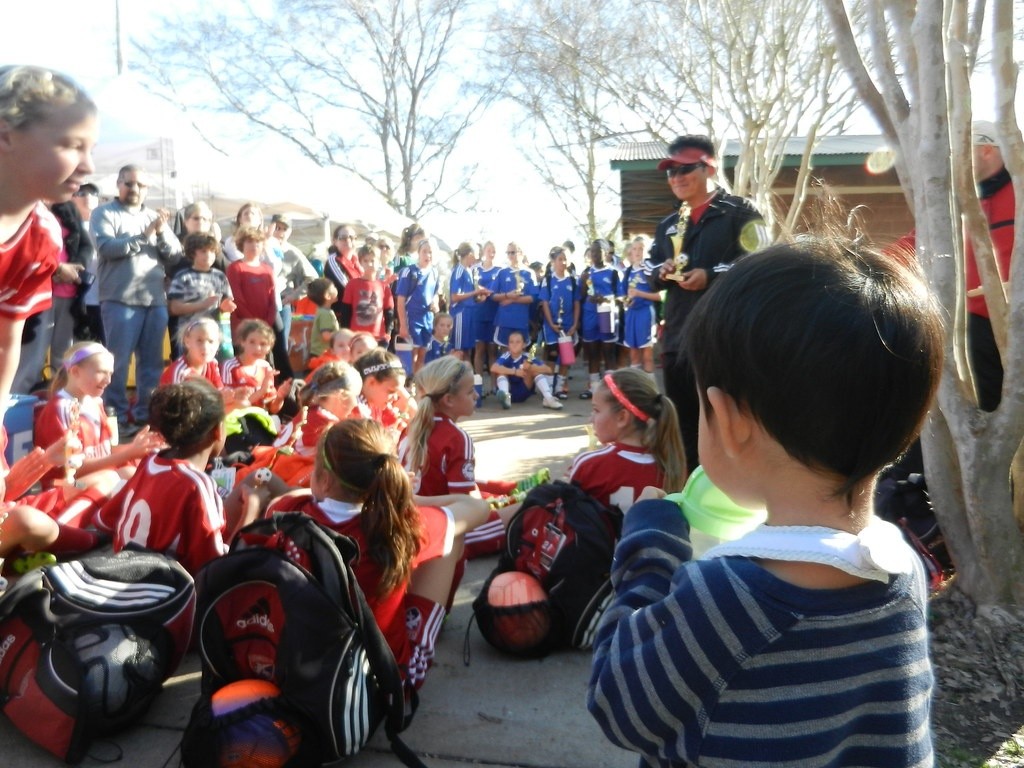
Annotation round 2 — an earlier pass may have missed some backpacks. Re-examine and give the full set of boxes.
[498,480,623,638]
[0,542,196,766]
[195,512,405,768]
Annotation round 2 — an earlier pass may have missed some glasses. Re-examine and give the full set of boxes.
[338,235,355,241]
[78,190,98,197]
[666,164,705,176]
[124,182,147,189]
[506,251,518,255]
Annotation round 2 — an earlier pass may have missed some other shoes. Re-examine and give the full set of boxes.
[543,400,563,409]
[579,390,592,399]
[497,390,511,408]
[509,468,550,497]
[554,391,568,400]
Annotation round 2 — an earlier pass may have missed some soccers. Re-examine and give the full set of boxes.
[485,571,552,644]
[201,675,301,764]
[70,623,163,716]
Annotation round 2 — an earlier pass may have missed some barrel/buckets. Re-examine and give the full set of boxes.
[559,331,576,366]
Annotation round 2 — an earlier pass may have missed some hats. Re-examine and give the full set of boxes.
[657,148,717,173]
[272,215,291,227]
[972,121,1000,146]
[80,181,99,192]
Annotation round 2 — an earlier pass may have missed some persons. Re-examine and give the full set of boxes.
[1,162,665,756]
[1,61,98,434]
[648,132,765,474]
[585,235,944,767]
[882,121,1015,412]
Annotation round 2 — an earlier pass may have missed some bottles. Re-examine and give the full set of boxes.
[105,405,119,447]
[660,464,768,561]
[486,468,551,511]
